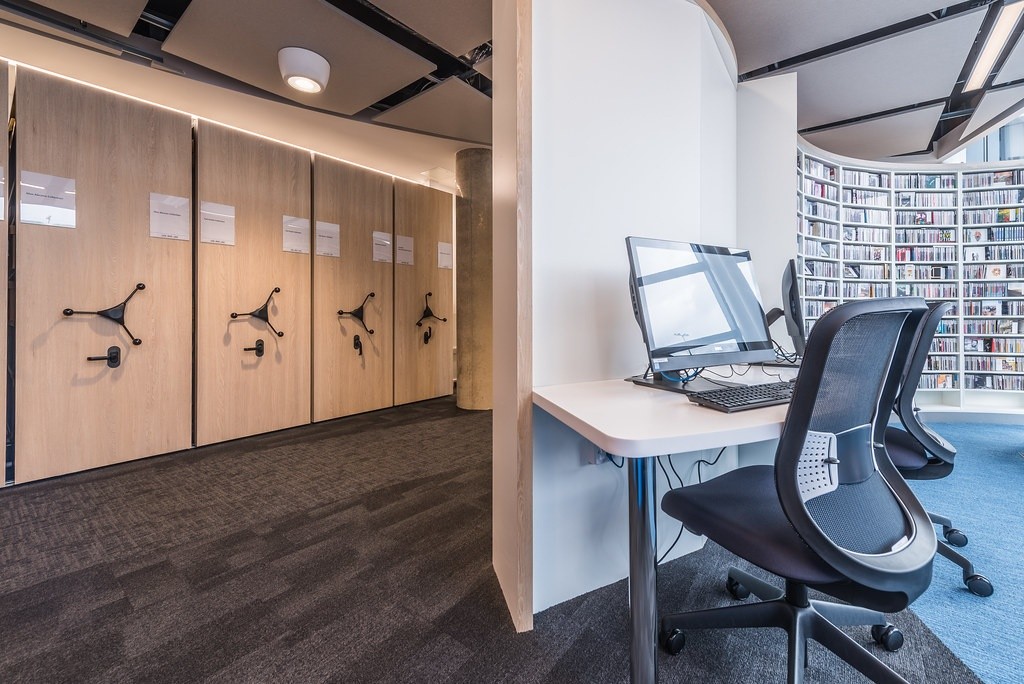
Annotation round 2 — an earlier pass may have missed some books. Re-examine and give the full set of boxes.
[794,152,1023,393]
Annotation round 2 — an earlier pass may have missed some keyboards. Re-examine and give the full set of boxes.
[684,381,795,414]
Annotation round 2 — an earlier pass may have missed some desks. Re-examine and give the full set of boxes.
[532,358,880,684]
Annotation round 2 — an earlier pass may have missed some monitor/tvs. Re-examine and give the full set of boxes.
[765,259,808,358]
[625,235,777,394]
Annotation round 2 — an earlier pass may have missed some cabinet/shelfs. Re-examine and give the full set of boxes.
[797,147,1024,414]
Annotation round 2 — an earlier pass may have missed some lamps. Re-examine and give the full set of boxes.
[277,47,331,95]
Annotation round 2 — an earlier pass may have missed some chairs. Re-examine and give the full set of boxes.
[888,300,994,598]
[658,296,938,683]
